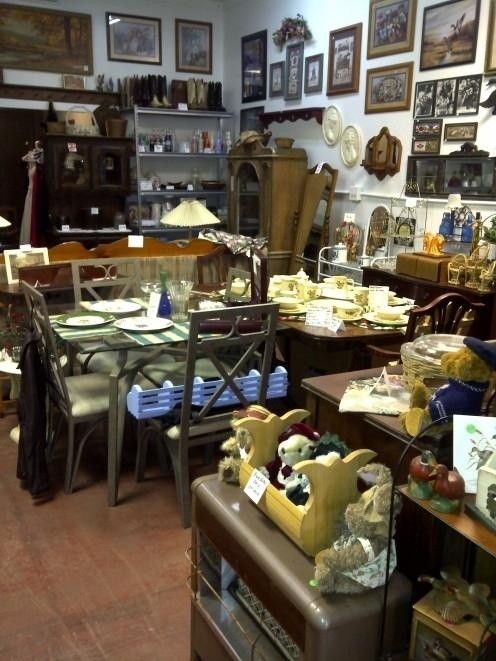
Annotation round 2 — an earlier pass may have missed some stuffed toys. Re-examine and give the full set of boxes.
[216,401,351,508]
[400,338,496,440]
[418,566,496,637]
[313,464,404,599]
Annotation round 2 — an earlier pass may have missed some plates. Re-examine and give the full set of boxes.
[362,312,411,327]
[220,284,364,322]
[113,318,174,332]
[91,302,142,314]
[339,126,362,168]
[57,312,115,327]
[322,105,342,147]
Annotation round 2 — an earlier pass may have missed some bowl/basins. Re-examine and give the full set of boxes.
[200,181,225,191]
[273,138,294,149]
[46,122,64,134]
[139,280,169,295]
[104,120,128,138]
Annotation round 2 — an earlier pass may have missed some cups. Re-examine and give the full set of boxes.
[56,217,69,230]
[150,196,228,229]
[168,280,194,321]
[331,276,355,293]
[353,286,369,306]
[414,236,424,253]
[368,286,389,311]
[112,212,126,230]
[152,177,160,192]
[12,346,21,362]
[360,257,370,266]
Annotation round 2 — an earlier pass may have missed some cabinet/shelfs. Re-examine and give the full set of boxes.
[374,410,493,656]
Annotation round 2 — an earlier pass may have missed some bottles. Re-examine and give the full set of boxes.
[140,128,233,155]
[449,170,481,187]
[296,268,309,282]
[158,272,172,316]
[439,209,496,258]
[191,168,198,192]
[331,244,347,263]
[45,98,57,131]
